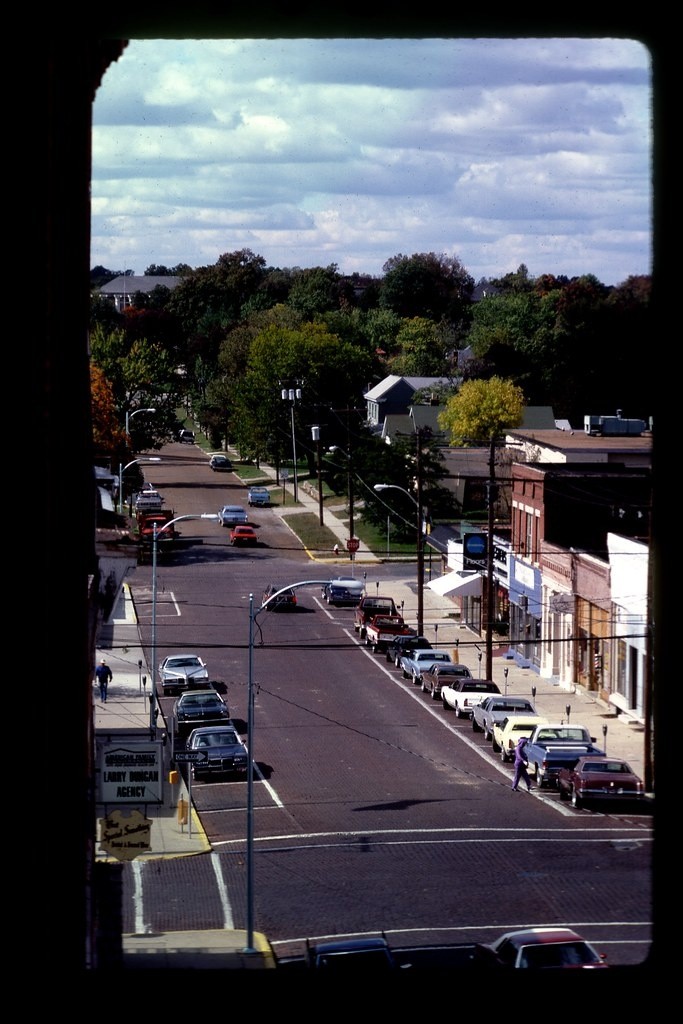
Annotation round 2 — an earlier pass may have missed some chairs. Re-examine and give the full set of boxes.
[222,736,234,744]
[197,737,210,746]
[185,700,196,704]
[205,698,216,703]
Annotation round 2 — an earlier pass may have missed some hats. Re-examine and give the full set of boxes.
[101,659,106,664]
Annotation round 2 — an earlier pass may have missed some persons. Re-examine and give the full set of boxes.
[512,737,535,792]
[95,659,113,703]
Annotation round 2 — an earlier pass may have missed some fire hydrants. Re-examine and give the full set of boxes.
[333,545,339,554]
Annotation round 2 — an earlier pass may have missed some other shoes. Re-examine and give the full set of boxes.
[512,788,520,792]
[527,787,536,791]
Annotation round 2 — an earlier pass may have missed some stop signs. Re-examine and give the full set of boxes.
[348,539,361,551]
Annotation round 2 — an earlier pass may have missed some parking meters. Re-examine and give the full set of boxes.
[363,571,607,753]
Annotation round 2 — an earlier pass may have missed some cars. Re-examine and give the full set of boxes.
[305,928,613,971]
[158,654,248,777]
[387,634,560,760]
[556,755,644,810]
[132,430,270,543]
[261,583,297,610]
[322,577,362,607]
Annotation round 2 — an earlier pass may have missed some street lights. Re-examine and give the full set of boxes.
[248,577,361,954]
[152,514,223,697]
[328,447,356,562]
[276,380,313,502]
[120,455,160,516]
[125,409,156,437]
[373,483,424,637]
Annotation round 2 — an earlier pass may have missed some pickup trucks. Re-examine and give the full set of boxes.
[523,724,603,787]
[352,595,415,652]
